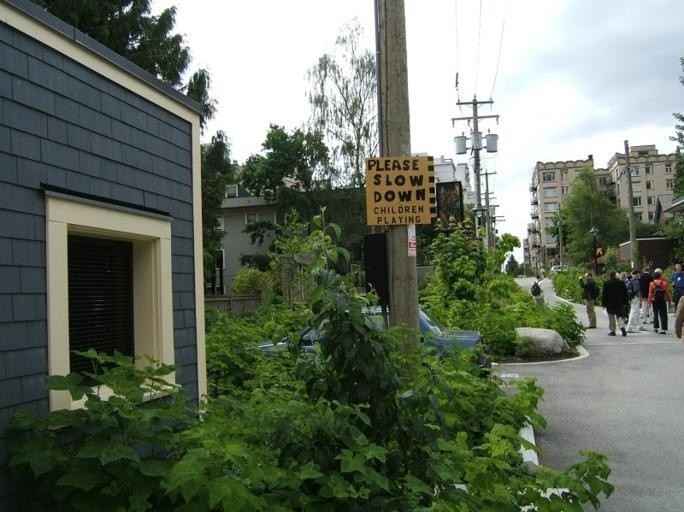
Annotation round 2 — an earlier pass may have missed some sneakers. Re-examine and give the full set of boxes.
[608,315,666,337]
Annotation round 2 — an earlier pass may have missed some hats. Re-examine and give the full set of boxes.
[632,270,642,275]
[654,268,663,274]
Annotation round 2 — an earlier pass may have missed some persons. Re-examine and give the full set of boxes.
[533,275,545,305]
[602,270,628,337]
[623,264,684,339]
[578,272,599,329]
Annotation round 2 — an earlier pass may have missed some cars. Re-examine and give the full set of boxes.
[551,265,571,273]
[246,305,490,378]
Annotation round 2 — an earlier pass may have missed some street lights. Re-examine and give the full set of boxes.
[453,126,498,238]
[589,226,600,274]
[472,201,487,287]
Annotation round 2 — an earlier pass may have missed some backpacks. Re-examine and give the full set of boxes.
[595,284,600,298]
[530,280,543,297]
[628,278,640,301]
[652,280,666,304]
